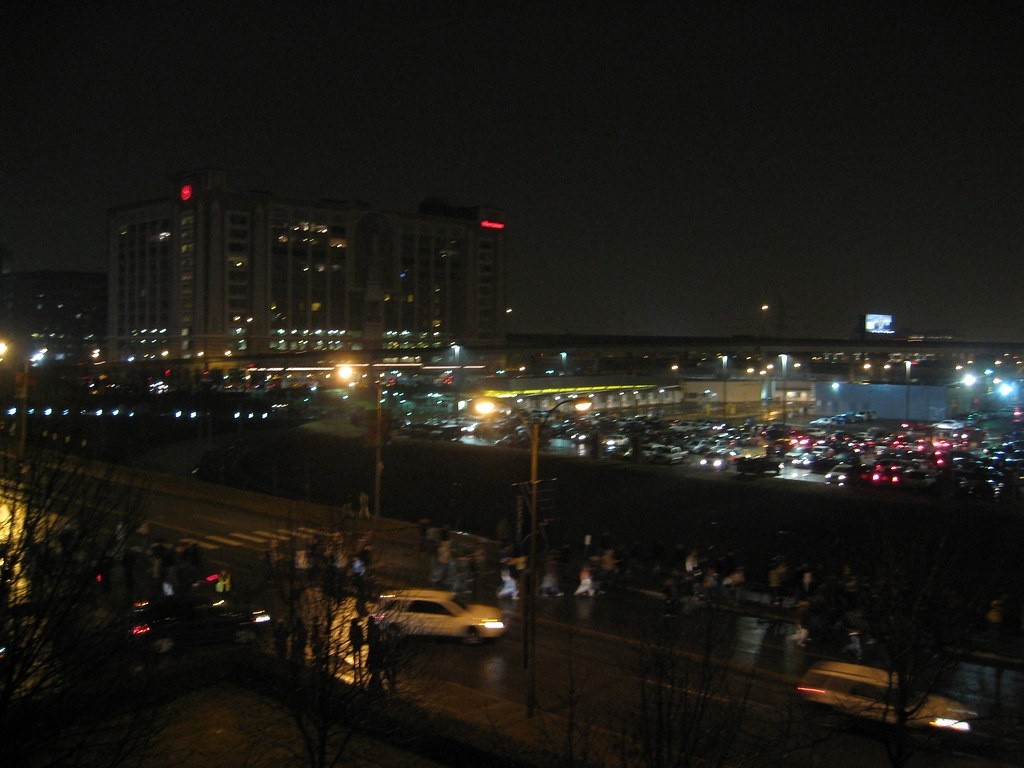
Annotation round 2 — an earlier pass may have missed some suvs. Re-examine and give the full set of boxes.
[797,662,977,740]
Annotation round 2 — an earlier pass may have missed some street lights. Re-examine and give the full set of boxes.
[333,357,383,522]
[0,339,28,461]
[466,394,594,734]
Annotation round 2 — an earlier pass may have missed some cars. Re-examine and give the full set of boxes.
[396,397,1024,501]
[374,590,509,644]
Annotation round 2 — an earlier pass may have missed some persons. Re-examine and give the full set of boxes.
[64,485,1013,687]
